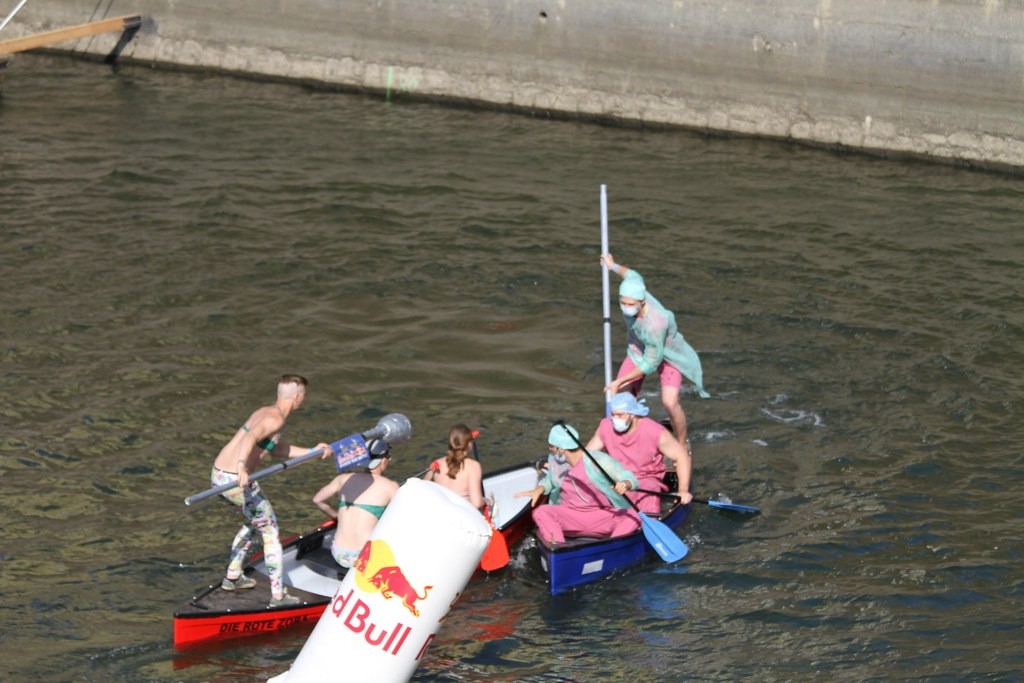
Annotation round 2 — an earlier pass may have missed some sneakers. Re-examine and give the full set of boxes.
[269,587,300,607]
[221,570,256,590]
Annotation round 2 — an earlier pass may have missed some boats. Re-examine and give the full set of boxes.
[532,416,693,596]
[173,452,552,650]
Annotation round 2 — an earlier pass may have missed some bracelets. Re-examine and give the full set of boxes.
[310,448,314,454]
[612,263,619,273]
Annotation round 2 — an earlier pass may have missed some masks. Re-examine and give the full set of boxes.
[619,304,642,317]
[611,415,632,432]
[554,454,566,465]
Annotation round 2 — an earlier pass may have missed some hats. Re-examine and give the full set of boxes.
[619,278,645,300]
[357,438,389,469]
[548,423,580,449]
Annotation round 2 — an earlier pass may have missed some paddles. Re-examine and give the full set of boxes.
[628,486,762,514]
[398,461,440,488]
[555,419,689,565]
[468,430,511,572]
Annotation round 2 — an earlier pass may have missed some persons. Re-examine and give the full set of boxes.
[514,424,642,543]
[585,392,693,520]
[313,439,399,569]
[210,375,332,607]
[423,424,494,520]
[600,253,711,445]
